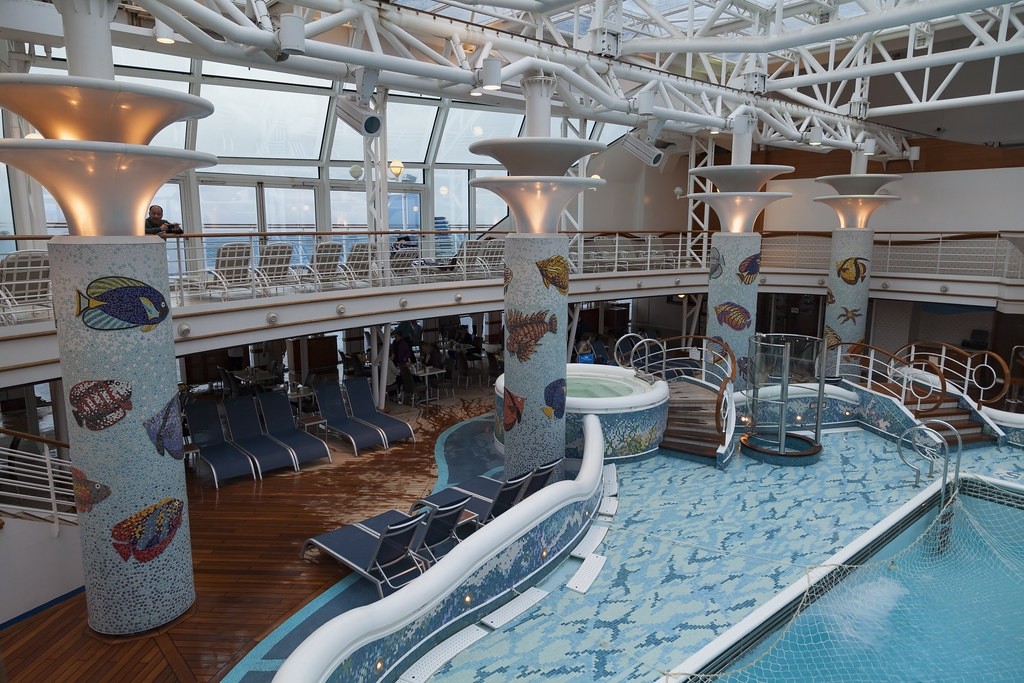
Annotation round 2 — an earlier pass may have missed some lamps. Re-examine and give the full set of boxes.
[638,90,654,117]
[710,127,720,135]
[618,118,667,168]
[470,89,482,97]
[335,67,382,138]
[673,186,687,200]
[349,165,365,185]
[279,13,305,56]
[806,126,822,146]
[153,17,176,45]
[482,58,502,90]
[861,138,876,155]
[387,160,405,182]
[906,146,920,162]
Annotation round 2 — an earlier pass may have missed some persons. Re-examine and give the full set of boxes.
[386,320,472,402]
[145,205,184,234]
[578,333,593,355]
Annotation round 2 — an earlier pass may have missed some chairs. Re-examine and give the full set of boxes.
[587,330,701,379]
[181,338,564,601]
[0,249,54,325]
[591,235,693,273]
[177,239,505,303]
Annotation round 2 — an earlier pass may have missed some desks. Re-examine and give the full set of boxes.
[231,368,279,390]
[296,415,328,445]
[288,386,315,419]
[457,509,480,532]
[183,444,201,483]
[448,346,476,371]
[410,367,446,404]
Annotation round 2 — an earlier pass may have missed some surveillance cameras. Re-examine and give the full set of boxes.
[621,131,664,166]
[336,95,383,137]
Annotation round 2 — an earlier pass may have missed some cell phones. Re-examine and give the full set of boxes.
[167,224,174,229]
